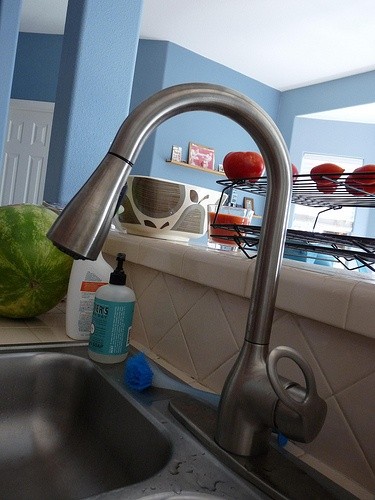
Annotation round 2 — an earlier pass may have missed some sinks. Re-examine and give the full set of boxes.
[1,342,206,500]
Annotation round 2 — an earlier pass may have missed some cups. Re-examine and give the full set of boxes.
[206,204,255,252]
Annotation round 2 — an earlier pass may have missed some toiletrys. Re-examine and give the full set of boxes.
[65,248,115,344]
[87,252,136,365]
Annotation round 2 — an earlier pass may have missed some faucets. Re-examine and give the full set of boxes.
[43,81,328,461]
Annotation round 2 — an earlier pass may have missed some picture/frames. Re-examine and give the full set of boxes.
[187,141,216,172]
[170,145,183,163]
[243,197,256,216]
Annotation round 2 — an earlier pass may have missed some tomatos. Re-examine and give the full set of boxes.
[223,151,265,185]
[310,163,343,183]
[345,164,375,196]
[292,164,298,184]
[317,181,337,193]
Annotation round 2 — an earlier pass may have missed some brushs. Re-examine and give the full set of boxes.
[124,352,287,448]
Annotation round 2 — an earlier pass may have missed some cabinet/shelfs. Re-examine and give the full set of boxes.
[210,171,375,273]
